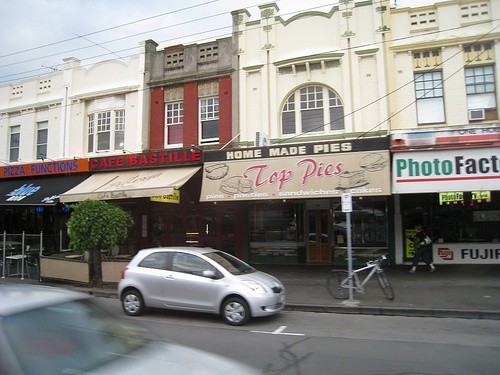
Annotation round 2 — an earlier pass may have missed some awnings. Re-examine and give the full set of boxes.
[59,165,203,203]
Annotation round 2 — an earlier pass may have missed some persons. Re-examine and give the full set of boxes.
[407,224,436,273]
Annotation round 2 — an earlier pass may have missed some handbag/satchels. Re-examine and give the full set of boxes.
[420,236,432,246]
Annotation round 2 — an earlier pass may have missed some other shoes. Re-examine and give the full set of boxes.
[408,270,416,274]
[430,267,438,272]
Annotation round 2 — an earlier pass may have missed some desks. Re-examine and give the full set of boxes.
[106,255,131,259]
[65,255,83,261]
[6,255,30,278]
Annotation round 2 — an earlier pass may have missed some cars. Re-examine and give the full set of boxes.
[118,246,287,326]
[0,282,262,374]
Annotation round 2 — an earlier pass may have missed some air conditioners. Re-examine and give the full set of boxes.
[469,109,485,120]
[254,131,265,147]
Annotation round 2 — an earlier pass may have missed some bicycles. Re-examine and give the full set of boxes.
[326,252,397,301]
